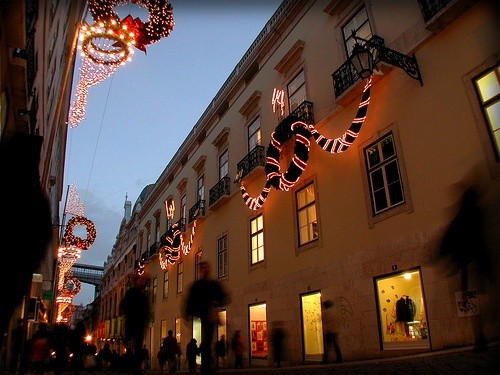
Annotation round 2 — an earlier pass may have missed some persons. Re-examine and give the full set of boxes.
[320,298,344,364]
[10,259,248,375]
[271,321,295,369]
[396,295,416,337]
